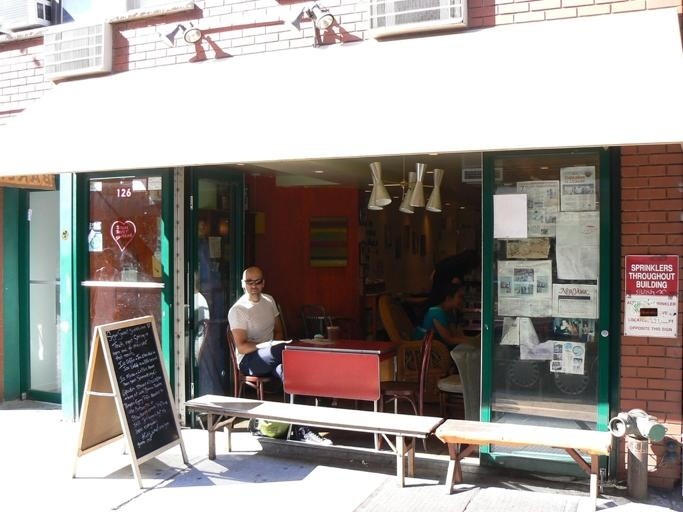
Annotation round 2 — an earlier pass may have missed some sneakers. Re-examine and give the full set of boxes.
[301,431,333,446]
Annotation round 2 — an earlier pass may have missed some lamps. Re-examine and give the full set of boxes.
[163,4,334,60]
[366,155,445,216]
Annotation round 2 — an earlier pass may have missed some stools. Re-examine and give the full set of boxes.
[439,375,465,420]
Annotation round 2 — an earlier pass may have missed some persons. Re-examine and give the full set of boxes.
[410,247,540,402]
[191,287,211,369]
[222,264,334,447]
[558,318,590,336]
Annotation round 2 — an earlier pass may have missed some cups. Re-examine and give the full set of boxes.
[326,324,340,343]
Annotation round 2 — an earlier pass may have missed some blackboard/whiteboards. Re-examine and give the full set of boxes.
[97,315,183,465]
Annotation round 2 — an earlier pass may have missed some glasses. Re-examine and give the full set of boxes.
[245,279,263,285]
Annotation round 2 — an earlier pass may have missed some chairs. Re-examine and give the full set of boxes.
[226,293,448,457]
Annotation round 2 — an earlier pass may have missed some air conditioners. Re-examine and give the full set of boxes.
[0,1,53,36]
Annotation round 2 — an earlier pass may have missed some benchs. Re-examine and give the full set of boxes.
[186,395,446,486]
[434,414,612,500]
